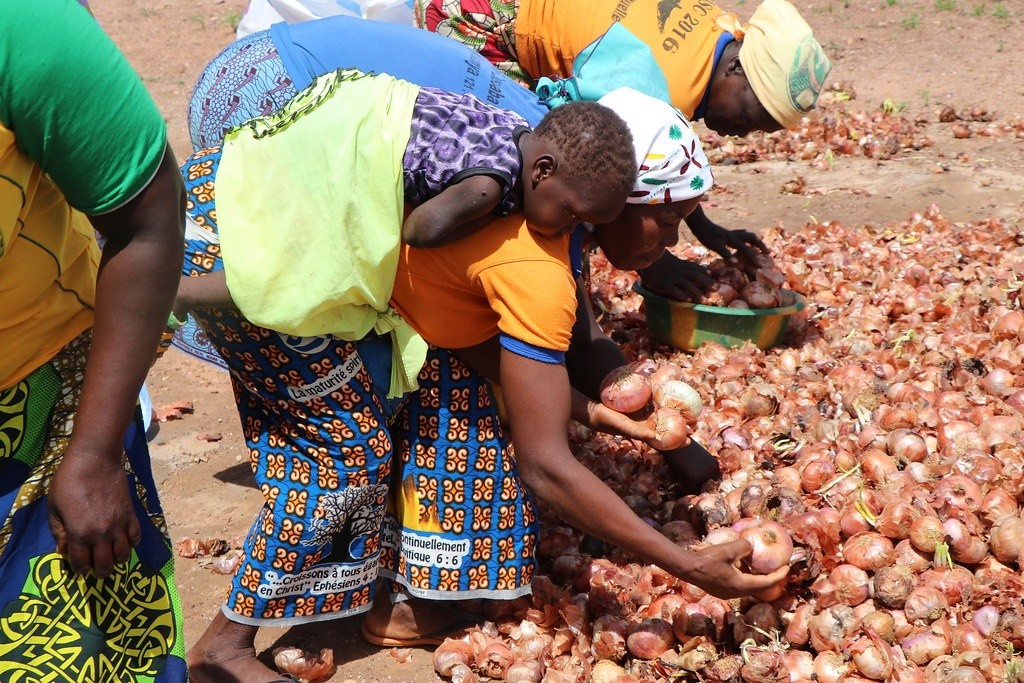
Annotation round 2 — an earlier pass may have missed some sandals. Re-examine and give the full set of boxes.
[146,308,189,368]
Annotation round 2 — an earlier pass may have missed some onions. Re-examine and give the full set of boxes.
[699,79,1024,165]
[428,212,1024,683]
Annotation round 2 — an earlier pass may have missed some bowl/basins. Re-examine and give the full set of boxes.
[631,278,806,352]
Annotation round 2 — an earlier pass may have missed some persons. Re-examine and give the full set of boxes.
[0,0,186,682]
[148,70,634,363]
[187,16,714,388]
[411,0,831,308]
[183,88,790,683]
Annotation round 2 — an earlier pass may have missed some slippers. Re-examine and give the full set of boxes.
[267,673,301,683]
[361,608,497,646]
